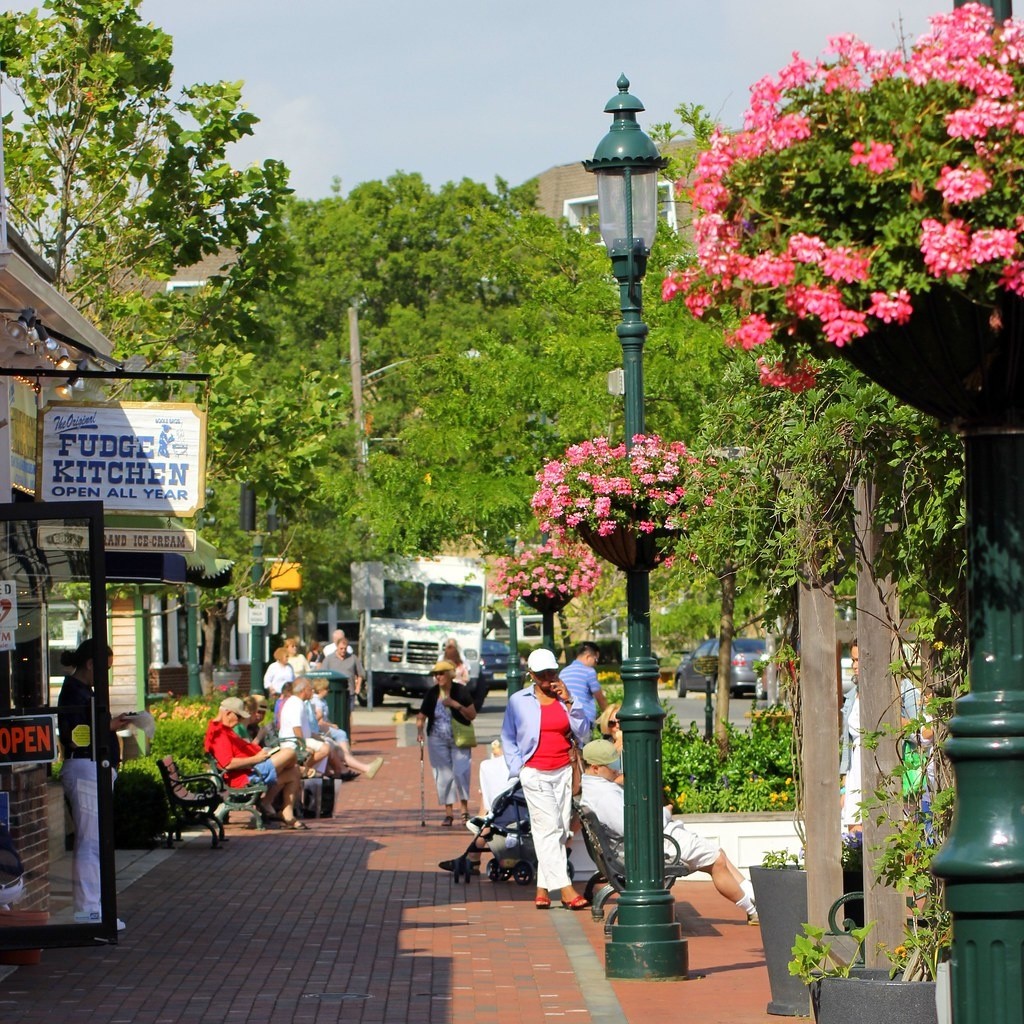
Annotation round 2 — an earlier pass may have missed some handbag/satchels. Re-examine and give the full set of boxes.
[568,746,586,795]
[452,718,477,748]
[297,778,335,819]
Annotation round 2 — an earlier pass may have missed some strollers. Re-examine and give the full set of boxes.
[455,782,574,885]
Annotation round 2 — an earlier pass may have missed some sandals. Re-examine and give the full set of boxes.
[438,854,481,876]
[441,816,453,827]
[295,764,316,779]
[279,817,309,830]
[461,813,470,825]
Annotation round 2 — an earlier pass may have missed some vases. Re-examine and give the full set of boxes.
[808,967,939,1024]
[0,909,49,965]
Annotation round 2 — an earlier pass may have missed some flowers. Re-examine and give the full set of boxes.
[528,429,727,570]
[486,538,603,609]
[657,0,1024,395]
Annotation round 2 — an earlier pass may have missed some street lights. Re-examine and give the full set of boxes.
[581,72,690,979]
[353,350,479,473]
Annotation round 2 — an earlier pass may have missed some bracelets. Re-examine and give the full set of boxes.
[565,697,574,705]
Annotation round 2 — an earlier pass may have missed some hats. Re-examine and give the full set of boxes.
[221,696,252,718]
[432,662,455,672]
[582,739,620,765]
[528,648,559,672]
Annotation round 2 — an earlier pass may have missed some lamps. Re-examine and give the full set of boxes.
[0,308,37,342]
[25,317,39,344]
[54,347,73,370]
[53,384,74,401]
[35,325,60,356]
[65,358,88,391]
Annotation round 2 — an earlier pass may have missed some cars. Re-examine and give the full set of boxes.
[755,641,855,701]
[477,640,525,691]
[674,637,766,698]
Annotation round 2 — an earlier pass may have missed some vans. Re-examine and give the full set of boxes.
[359,555,485,707]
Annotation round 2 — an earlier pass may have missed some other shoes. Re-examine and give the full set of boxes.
[80,917,126,931]
[746,898,760,927]
[365,757,383,778]
[335,769,361,780]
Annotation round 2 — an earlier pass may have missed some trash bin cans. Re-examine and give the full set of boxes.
[304,670,351,749]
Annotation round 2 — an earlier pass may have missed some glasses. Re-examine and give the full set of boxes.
[590,654,599,663]
[433,671,448,676]
[608,720,621,728]
[534,670,555,678]
[234,712,242,720]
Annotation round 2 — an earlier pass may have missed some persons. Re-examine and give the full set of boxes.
[322,638,366,745]
[275,676,384,782]
[263,647,295,698]
[292,634,306,659]
[438,783,531,876]
[556,642,604,765]
[840,637,941,873]
[317,629,354,687]
[58,639,132,930]
[580,739,761,926]
[306,640,320,663]
[501,648,588,910]
[281,638,310,676]
[205,694,309,830]
[600,704,627,772]
[416,640,477,826]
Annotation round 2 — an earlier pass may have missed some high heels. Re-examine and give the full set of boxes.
[535,897,551,909]
[561,896,588,910]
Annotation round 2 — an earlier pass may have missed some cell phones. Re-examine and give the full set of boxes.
[270,746,280,755]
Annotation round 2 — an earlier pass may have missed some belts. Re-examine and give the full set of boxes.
[64,752,92,758]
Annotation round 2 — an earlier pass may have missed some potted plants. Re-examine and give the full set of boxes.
[748,835,862,1017]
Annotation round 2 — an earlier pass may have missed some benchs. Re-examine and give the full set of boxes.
[572,794,690,940]
[204,751,271,831]
[265,723,310,766]
[156,754,230,851]
